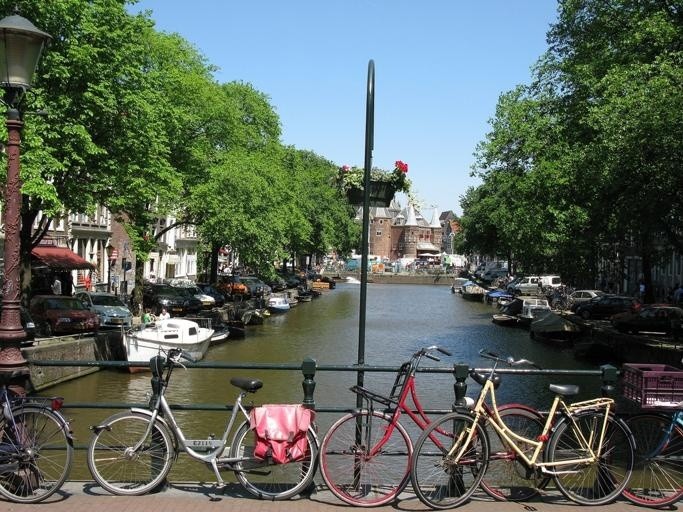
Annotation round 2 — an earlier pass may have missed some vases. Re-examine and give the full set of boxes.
[345,181,396,207]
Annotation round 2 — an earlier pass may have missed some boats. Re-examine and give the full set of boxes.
[246,277,341,314]
[452,277,592,340]
[103,302,283,368]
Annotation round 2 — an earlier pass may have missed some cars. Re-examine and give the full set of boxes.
[29,280,215,330]
[569,287,683,339]
[216,265,320,291]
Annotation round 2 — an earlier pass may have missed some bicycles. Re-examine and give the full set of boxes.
[0,367,74,501]
[601,362,683,504]
[548,287,571,311]
[408,347,634,503]
[87,358,320,499]
[319,344,551,505]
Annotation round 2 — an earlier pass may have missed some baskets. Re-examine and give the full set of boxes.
[622,363,683,409]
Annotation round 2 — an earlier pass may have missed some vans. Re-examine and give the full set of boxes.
[479,259,558,294]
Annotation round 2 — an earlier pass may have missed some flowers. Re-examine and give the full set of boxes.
[335,160,422,217]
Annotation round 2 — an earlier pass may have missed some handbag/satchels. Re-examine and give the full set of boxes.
[250,404,310,465]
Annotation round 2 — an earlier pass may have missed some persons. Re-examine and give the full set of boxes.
[631,299,641,314]
[661,285,683,308]
[156,308,170,323]
[143,308,155,326]
[256,286,264,310]
[50,276,62,295]
[214,276,233,298]
[639,280,645,298]
[64,271,73,296]
